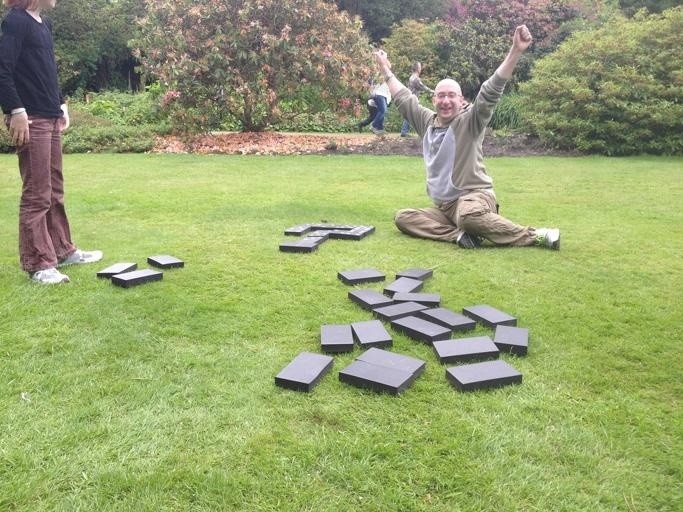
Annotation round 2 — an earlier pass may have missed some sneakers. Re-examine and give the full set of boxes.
[29,267,71,286]
[57,249,103,265]
[455,230,484,249]
[541,227,560,249]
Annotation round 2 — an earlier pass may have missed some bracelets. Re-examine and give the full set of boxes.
[369,82,391,134]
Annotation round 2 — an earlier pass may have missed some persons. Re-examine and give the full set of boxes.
[358,82,382,133]
[0,0,103,283]
[377,24,561,250]
[400,62,435,137]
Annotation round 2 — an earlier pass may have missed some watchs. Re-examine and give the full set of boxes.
[384,73,394,82]
